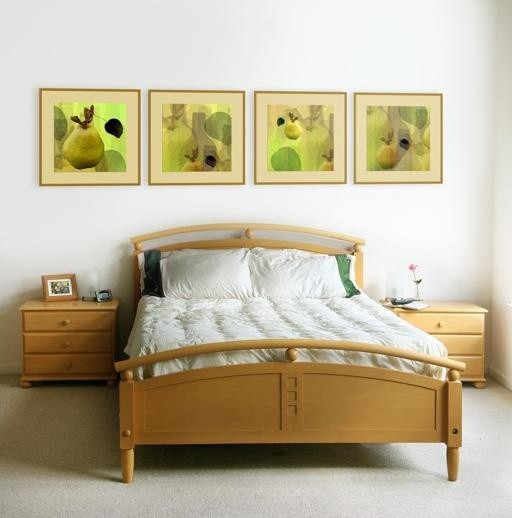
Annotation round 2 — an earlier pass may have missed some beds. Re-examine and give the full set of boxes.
[111,222,468,484]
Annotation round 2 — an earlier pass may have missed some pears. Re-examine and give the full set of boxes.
[268,104,333,171]
[162,104,231,172]
[366,106,430,172]
[54,104,128,172]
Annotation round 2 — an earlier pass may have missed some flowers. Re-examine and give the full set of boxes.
[409,262,423,296]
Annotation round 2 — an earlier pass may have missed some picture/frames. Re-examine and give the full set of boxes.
[38,87,142,186]
[146,88,248,187]
[253,89,347,186]
[350,88,445,186]
[39,271,79,302]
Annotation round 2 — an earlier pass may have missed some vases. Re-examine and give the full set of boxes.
[412,283,424,300]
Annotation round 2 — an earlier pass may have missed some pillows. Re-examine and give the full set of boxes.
[158,248,250,301]
[336,252,363,299]
[251,245,348,300]
[135,248,167,298]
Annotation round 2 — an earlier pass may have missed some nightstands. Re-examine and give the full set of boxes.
[16,298,120,390]
[383,298,492,391]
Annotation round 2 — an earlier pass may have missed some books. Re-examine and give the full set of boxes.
[382,298,430,311]
